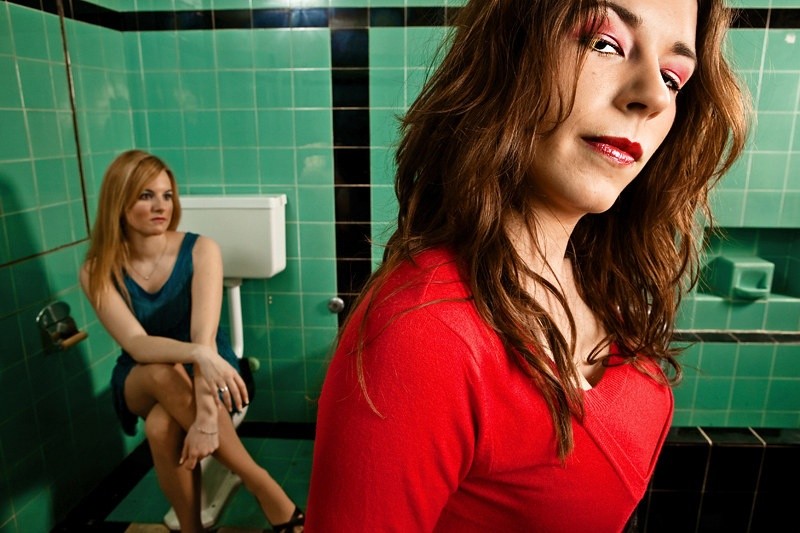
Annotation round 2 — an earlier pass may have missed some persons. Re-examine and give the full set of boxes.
[79,149,308,533]
[303,0,754,533]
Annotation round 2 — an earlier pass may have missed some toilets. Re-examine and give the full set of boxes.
[162,193,287,530]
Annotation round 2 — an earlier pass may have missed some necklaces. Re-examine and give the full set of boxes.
[129,233,168,281]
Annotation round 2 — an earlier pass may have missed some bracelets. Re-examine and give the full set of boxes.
[194,423,219,437]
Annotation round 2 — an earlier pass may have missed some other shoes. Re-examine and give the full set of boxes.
[256,486,306,533]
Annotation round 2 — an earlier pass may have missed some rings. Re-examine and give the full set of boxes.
[219,387,228,392]
